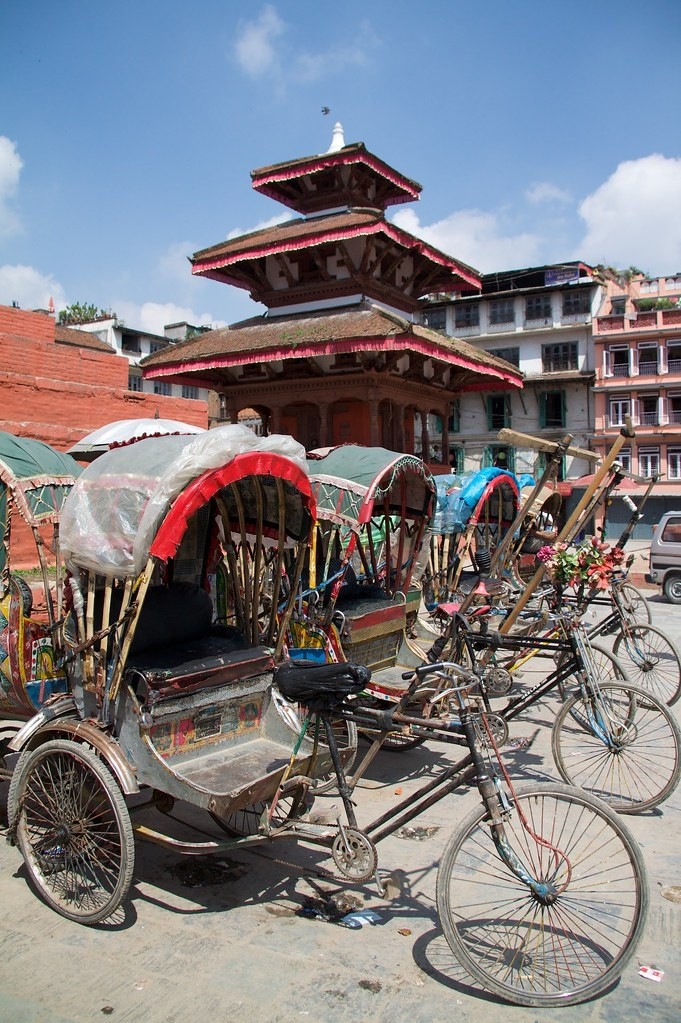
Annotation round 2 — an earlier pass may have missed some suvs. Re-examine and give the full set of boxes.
[644,511,681,605]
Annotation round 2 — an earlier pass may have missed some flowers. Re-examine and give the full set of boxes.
[536,537,633,608]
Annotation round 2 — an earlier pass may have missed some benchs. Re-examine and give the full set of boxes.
[489,543,537,568]
[85,579,277,705]
[273,555,409,643]
[434,555,507,599]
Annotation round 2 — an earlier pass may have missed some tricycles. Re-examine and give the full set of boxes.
[0,415,681,1011]
